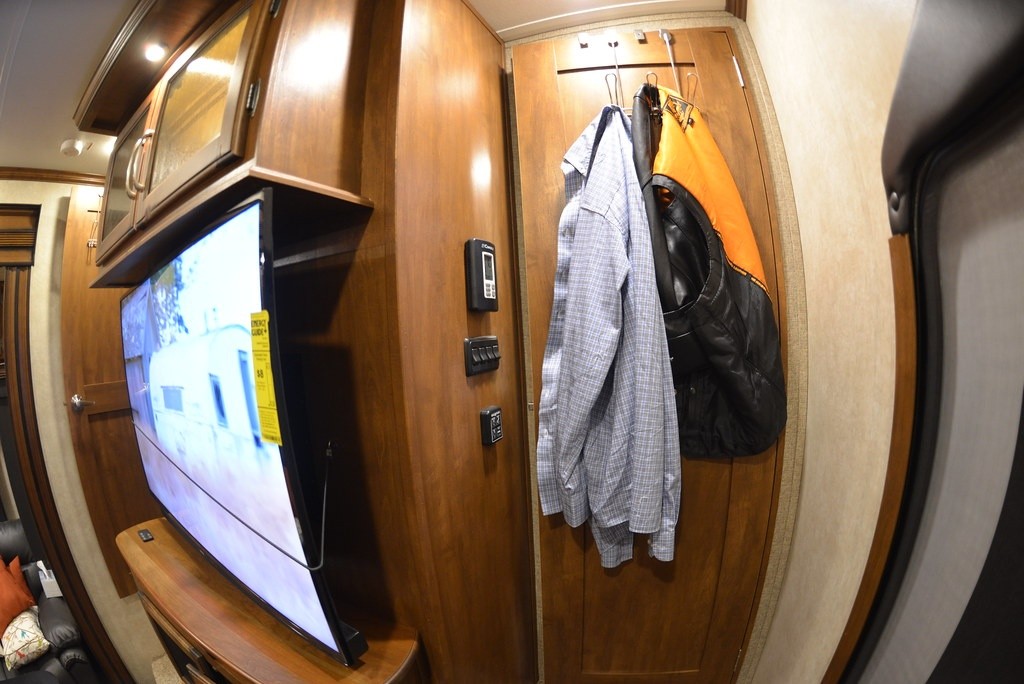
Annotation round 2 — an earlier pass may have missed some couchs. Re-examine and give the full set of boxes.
[0,519,94,684]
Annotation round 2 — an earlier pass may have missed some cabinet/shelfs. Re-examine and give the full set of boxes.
[116,515,432,684]
[71,0,371,290]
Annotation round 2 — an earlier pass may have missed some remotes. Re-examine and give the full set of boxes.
[138,528,153,542]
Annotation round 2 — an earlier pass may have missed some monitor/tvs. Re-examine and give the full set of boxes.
[119,186,369,668]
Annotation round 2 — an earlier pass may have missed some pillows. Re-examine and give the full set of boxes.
[0,555,34,639]
[6,556,36,606]
[2,606,50,671]
